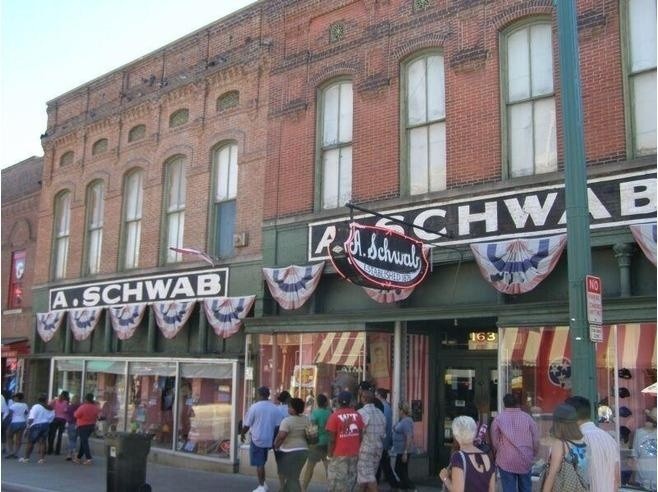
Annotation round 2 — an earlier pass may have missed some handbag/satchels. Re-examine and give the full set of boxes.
[304,420,319,443]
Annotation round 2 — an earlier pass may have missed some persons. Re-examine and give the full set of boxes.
[356,391,386,492]
[1,394,10,441]
[64,395,81,463]
[37,439,46,455]
[302,394,334,492]
[633,407,658,492]
[47,391,73,456]
[325,391,363,492]
[5,392,29,460]
[439,416,496,492]
[274,398,312,492]
[450,415,494,464]
[19,392,55,463]
[358,385,384,492]
[3,395,16,443]
[181,397,195,441]
[540,406,591,492]
[375,388,398,492]
[273,391,291,492]
[241,387,281,492]
[392,402,417,492]
[73,394,97,465]
[101,391,120,433]
[491,394,541,492]
[565,396,620,492]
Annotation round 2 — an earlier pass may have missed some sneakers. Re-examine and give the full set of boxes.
[4,454,13,458]
[38,459,46,463]
[252,485,265,492]
[12,454,17,459]
[83,459,95,464]
[73,457,80,463]
[262,482,268,489]
[19,458,29,464]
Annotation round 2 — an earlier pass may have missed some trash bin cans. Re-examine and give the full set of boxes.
[104,431,153,492]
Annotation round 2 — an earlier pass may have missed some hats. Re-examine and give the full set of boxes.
[338,389,352,400]
[37,392,47,401]
[553,403,576,422]
[646,407,657,420]
[621,407,630,416]
[619,388,629,397]
[618,369,631,377]
